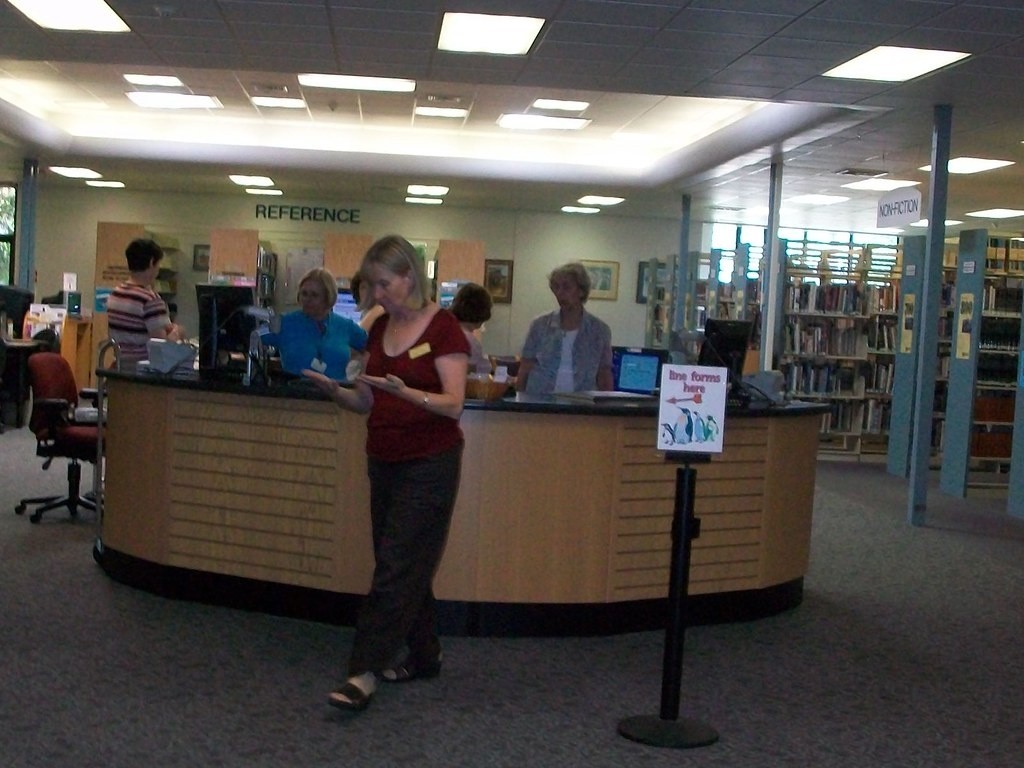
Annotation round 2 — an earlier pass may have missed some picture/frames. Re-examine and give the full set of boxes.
[636,261,679,304]
[193,244,211,271]
[576,259,620,300]
[483,259,514,304]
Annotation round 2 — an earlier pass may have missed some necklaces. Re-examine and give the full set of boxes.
[387,307,428,334]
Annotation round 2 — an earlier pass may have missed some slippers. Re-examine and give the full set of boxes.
[383,654,442,683]
[329,670,379,709]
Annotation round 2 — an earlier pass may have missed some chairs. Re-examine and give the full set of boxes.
[14,352,107,524]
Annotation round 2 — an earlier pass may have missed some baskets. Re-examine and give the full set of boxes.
[466,380,510,401]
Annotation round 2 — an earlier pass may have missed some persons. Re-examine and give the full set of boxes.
[346,269,384,334]
[511,260,615,395]
[299,231,472,712]
[276,265,369,386]
[443,281,509,399]
[104,237,186,368]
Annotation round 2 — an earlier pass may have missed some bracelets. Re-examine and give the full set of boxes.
[419,390,429,409]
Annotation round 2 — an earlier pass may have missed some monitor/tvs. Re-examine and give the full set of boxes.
[42,290,82,316]
[699,318,752,393]
[610,347,669,395]
[195,284,257,354]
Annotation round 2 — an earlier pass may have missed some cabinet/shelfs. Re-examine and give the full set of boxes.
[886,234,959,480]
[431,239,486,344]
[22,309,93,396]
[936,228,1024,501]
[90,222,180,389]
[759,239,902,463]
[644,243,764,366]
[323,233,373,289]
[209,229,278,332]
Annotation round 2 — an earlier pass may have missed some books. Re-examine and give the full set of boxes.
[644,234,1023,469]
